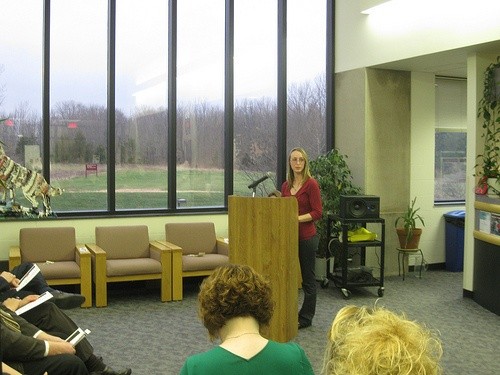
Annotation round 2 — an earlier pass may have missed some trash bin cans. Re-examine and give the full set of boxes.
[443,209,466,272]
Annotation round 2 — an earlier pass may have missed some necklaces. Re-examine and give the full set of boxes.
[223,332,259,340]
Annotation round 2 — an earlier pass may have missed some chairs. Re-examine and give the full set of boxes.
[156,223,229,300]
[9,227,92,308]
[86,225,171,307]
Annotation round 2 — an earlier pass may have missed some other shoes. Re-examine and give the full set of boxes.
[49,289,86,308]
[298,315,312,328]
[91,365,132,375]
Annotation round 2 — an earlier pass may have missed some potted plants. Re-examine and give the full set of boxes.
[473,55,500,198]
[395,197,425,249]
[308,147,363,257]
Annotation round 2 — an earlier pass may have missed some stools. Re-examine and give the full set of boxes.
[397,248,424,280]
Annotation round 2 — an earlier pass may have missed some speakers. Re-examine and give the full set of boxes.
[339,195,380,218]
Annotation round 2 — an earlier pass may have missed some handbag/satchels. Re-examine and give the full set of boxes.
[338,225,377,241]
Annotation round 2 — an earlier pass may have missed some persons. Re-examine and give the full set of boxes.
[0,264,132,375]
[268,191,281,197]
[321,304,443,375]
[180,262,314,375]
[281,147,323,329]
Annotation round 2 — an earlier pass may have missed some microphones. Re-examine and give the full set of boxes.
[248,171,273,188]
